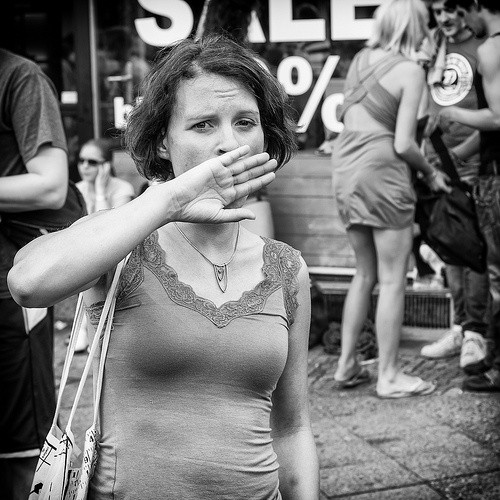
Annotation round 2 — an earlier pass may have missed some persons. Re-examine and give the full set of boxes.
[331,0,452,401]
[0,45,68,500]
[7,33,320,500]
[75,140,133,215]
[421,0,500,393]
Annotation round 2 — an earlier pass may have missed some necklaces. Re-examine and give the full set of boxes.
[174,221,240,294]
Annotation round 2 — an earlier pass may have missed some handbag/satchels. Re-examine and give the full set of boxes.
[27,426,101,500]
[0,166,87,249]
[414,114,486,273]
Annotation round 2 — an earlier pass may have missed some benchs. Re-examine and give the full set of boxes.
[111,153,450,295]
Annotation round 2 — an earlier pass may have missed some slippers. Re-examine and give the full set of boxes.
[378,374,437,399]
[338,366,373,388]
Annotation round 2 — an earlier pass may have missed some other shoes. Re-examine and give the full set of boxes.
[460,367,500,391]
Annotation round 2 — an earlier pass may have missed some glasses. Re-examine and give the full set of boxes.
[77,158,103,166]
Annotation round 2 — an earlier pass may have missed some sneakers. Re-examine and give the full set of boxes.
[460,330,488,368]
[420,324,463,358]
